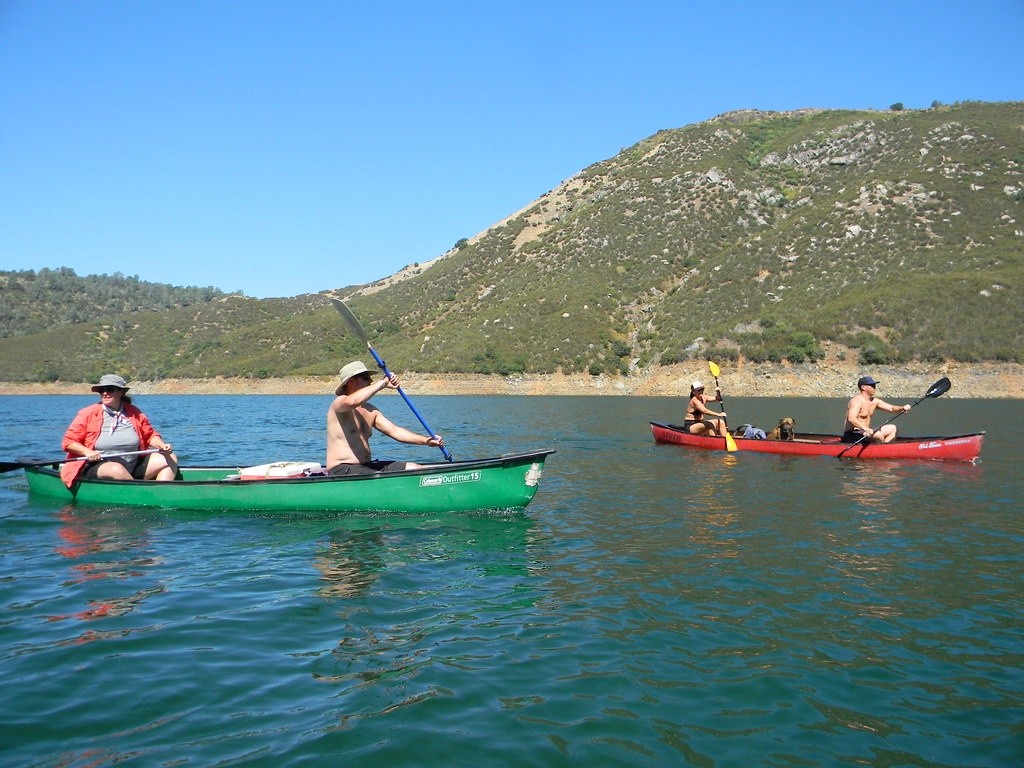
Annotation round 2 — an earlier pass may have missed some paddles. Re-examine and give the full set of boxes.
[0,446,166,475]
[329,297,453,464]
[708,360,738,453]
[837,376,951,459]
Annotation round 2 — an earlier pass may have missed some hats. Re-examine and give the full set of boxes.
[91,374,131,391]
[858,376,880,388]
[691,381,706,392]
[335,360,379,396]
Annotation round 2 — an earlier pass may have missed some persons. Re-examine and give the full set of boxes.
[684,381,728,437]
[62,374,178,482]
[844,376,911,444]
[326,361,445,477]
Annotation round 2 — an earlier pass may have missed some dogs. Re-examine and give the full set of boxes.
[766,417,796,442]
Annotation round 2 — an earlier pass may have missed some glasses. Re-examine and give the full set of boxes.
[98,386,120,394]
[697,388,704,392]
[354,373,370,381]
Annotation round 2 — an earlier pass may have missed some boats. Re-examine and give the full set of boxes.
[651,407,988,460]
[14,443,559,518]
